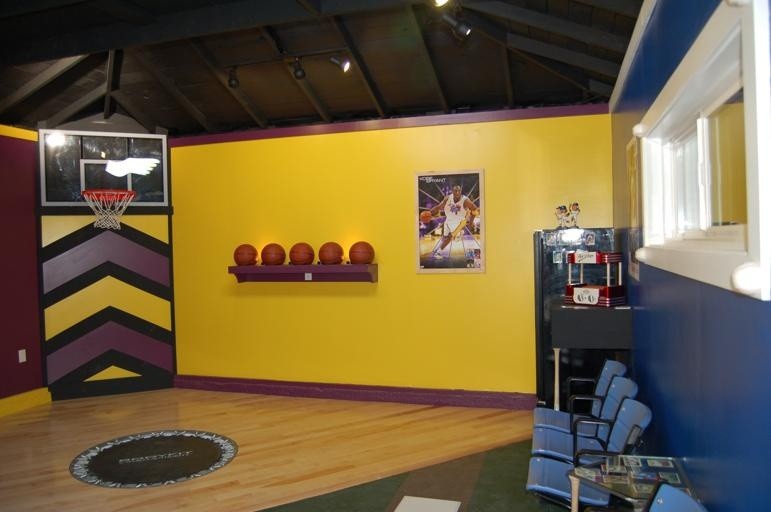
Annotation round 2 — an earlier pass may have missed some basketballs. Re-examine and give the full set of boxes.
[262,244,286,264]
[234,244,258,265]
[350,242,375,264]
[421,211,433,223]
[290,243,315,265]
[319,242,344,265]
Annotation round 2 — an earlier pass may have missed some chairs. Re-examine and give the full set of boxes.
[524,360,711,512]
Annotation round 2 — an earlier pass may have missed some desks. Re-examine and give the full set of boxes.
[551,295,633,413]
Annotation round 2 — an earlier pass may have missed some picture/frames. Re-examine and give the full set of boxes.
[415,168,485,274]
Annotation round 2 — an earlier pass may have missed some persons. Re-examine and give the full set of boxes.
[431,186,478,258]
[555,202,580,228]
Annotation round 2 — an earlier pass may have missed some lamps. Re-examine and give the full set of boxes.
[440,10,472,42]
[223,47,353,87]
